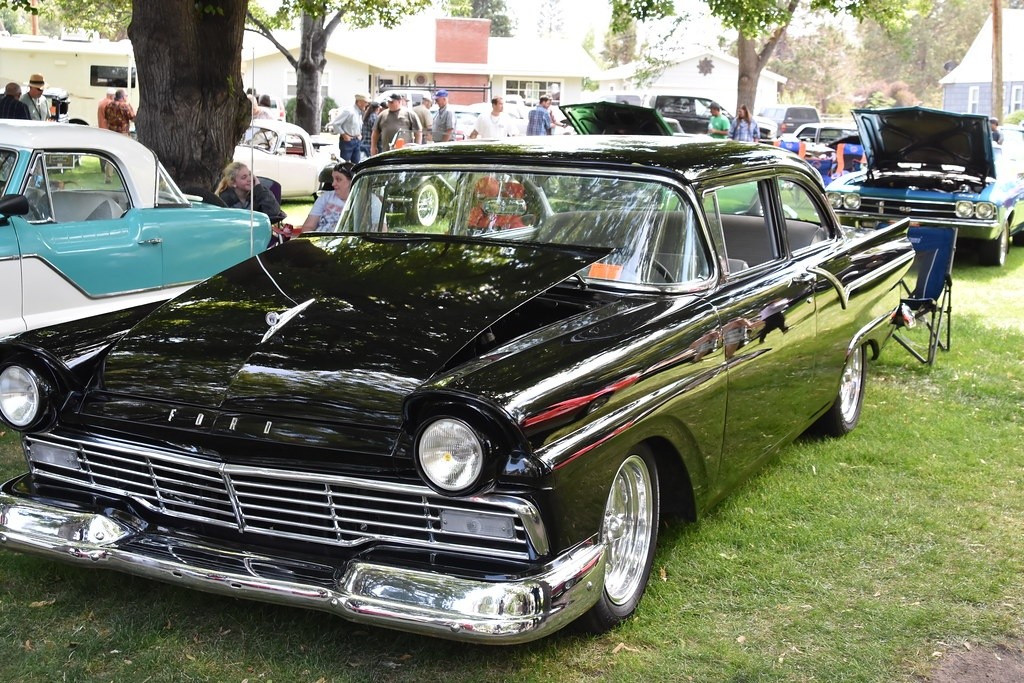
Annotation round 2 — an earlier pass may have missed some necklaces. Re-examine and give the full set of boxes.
[27,92,42,120]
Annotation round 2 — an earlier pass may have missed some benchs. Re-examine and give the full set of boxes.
[534,210,822,283]
[286,147,304,156]
[37,190,125,221]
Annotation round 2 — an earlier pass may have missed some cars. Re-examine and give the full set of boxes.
[1,124,918,646]
[824,105,1024,268]
[0,114,274,338]
[230,87,868,232]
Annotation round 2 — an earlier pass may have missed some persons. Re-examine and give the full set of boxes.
[526,96,567,136]
[0,82,32,120]
[302,162,388,233]
[730,104,760,144]
[990,118,1003,145]
[707,103,730,139]
[20,74,57,122]
[469,97,519,139]
[98,89,136,137]
[243,88,275,144]
[215,161,288,226]
[690,297,794,364]
[332,89,457,165]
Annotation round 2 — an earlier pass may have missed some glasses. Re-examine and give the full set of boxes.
[32,87,43,91]
[333,164,353,176]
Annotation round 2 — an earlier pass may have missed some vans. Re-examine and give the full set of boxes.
[0,31,140,136]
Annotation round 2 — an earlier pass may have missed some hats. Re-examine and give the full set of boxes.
[707,102,720,109]
[431,90,448,99]
[24,74,49,89]
[355,94,372,103]
[105,87,116,95]
[384,93,402,101]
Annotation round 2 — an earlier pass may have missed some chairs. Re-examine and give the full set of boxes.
[776,139,867,210]
[257,177,283,248]
[876,222,958,370]
